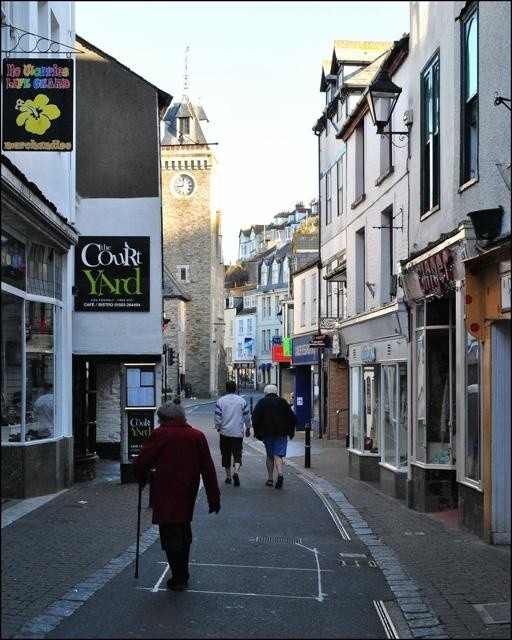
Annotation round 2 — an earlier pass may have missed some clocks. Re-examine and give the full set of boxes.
[169,172,196,200]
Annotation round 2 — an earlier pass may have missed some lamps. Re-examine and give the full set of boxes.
[362,68,413,158]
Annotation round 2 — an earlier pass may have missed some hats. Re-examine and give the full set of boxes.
[157,398,185,420]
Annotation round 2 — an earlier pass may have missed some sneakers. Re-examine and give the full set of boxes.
[265,480,273,486]
[233,473,240,486]
[275,475,284,488]
[167,579,187,591]
[225,478,232,484]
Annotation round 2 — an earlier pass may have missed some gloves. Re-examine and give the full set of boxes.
[208,502,221,514]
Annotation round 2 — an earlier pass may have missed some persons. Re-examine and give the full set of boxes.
[132,401,223,593]
[31,382,54,439]
[10,390,33,424]
[251,384,295,491]
[215,380,252,487]
